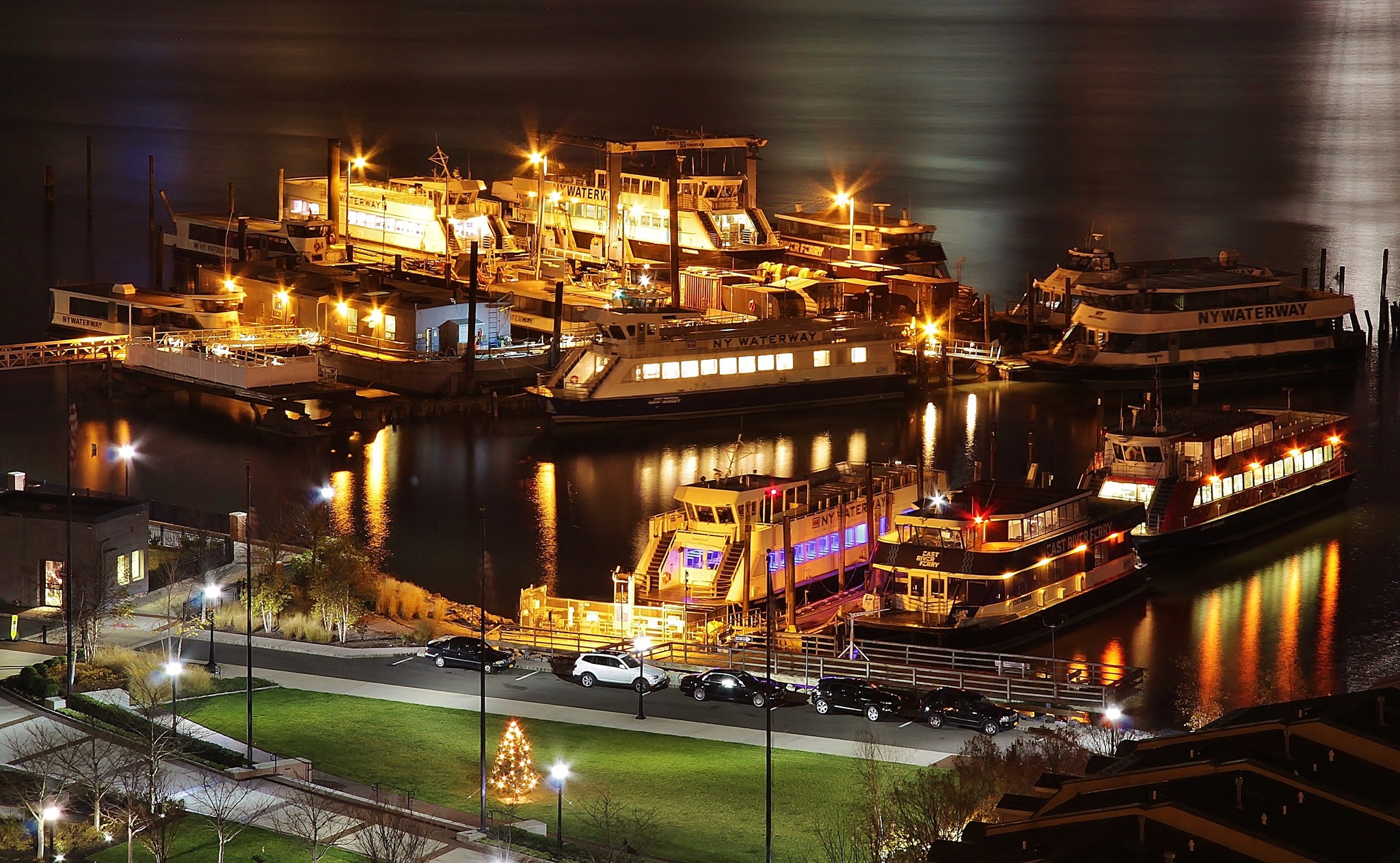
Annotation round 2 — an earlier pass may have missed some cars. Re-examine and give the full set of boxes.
[678,668,786,708]
[424,635,516,675]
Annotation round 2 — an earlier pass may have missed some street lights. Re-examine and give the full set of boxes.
[119,445,133,502]
[633,636,651,720]
[321,487,334,534]
[204,583,222,675]
[166,662,183,748]
[44,805,61,861]
[551,761,569,863]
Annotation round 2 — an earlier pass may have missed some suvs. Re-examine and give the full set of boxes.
[811,677,902,722]
[917,687,1019,736]
[572,651,667,692]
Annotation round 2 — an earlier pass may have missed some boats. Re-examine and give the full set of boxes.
[42,276,247,350]
[146,131,998,360]
[999,220,1359,372]
[1075,354,1361,540]
[834,427,1149,634]
[520,289,911,426]
[610,433,952,620]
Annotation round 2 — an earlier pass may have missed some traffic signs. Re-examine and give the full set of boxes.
[734,635,752,643]
